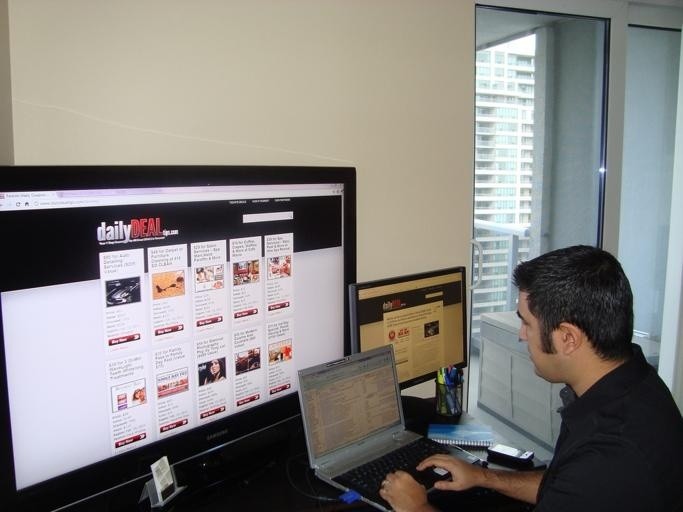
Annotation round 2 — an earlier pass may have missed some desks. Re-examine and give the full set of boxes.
[188,395,546,511]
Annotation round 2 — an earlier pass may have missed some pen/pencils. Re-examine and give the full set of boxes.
[434,365,464,415]
[447,444,490,467]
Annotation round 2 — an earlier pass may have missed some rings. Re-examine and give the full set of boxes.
[380,479,387,488]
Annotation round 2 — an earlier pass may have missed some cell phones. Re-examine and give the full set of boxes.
[488,444,534,464]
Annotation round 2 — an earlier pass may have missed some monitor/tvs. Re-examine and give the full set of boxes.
[0,166,358,512]
[349,265,467,425]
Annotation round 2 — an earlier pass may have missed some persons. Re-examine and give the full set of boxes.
[377,241,682,511]
[203,358,226,385]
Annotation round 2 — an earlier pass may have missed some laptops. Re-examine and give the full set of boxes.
[299,345,479,512]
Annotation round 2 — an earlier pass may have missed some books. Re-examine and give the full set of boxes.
[425,422,495,447]
